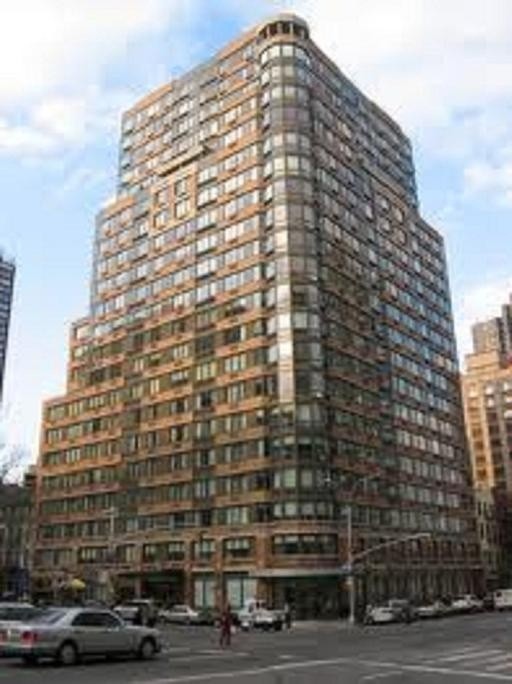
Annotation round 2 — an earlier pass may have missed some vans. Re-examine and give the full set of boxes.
[112,599,161,622]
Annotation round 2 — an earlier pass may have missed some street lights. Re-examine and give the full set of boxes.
[326,471,383,625]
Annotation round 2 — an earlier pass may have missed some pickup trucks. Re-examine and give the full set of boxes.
[236,600,286,631]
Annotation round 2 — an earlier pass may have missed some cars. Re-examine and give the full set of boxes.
[7,606,161,666]
[0,601,38,655]
[159,605,208,625]
[363,587,512,626]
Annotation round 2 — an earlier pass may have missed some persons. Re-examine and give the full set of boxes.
[219,605,233,648]
